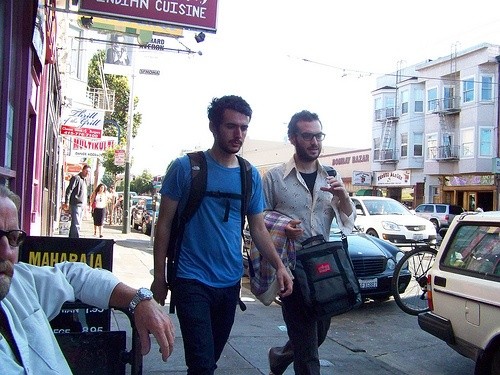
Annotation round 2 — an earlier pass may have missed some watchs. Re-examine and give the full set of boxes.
[127,287,153,313]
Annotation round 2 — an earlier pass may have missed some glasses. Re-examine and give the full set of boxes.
[0,230,27,247]
[295,130,326,141]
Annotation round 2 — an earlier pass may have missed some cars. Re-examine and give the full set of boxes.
[350,196,437,251]
[417,210,500,375]
[242,216,412,302]
[116,192,161,236]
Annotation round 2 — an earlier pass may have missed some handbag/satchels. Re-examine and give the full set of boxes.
[293,235,362,321]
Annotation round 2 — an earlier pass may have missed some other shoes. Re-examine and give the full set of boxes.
[269,348,275,375]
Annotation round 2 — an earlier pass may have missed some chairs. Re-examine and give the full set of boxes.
[49,301,143,375]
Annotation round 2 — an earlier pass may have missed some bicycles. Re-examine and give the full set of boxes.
[391,228,449,316]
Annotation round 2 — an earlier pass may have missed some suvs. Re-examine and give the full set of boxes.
[412,204,463,233]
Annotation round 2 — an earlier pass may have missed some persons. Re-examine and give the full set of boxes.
[109,186,124,225]
[261,107,357,375]
[372,203,387,215]
[0,186,176,375]
[91,183,107,238]
[150,94,295,375]
[62,165,92,238]
